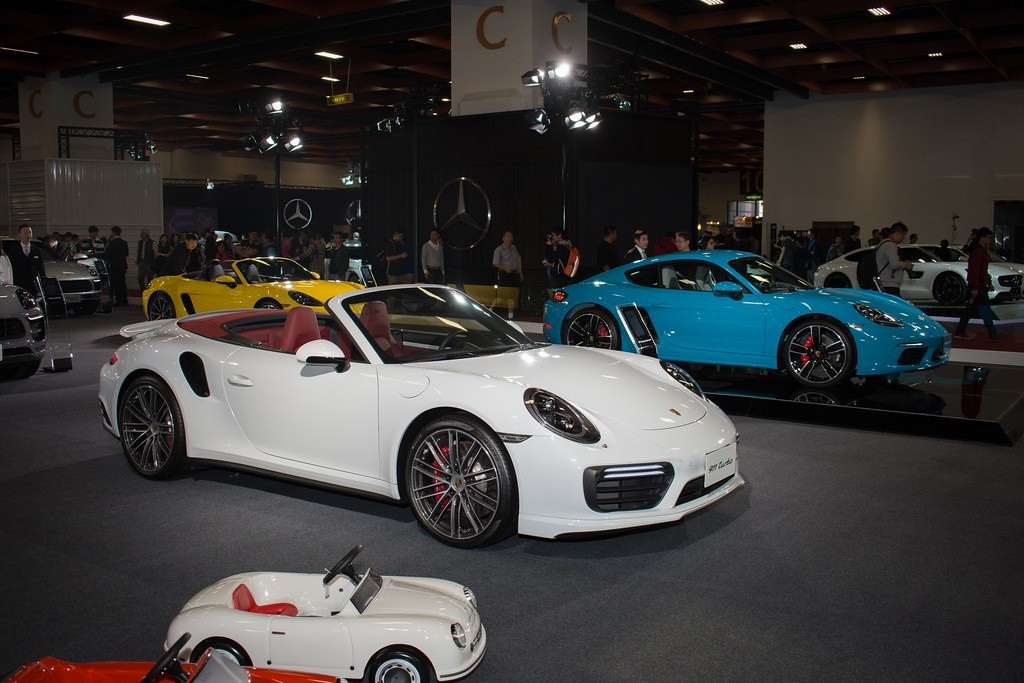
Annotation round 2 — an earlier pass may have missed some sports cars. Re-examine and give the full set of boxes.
[541,249,952,387]
[812,244,1023,305]
[96,283,745,550]
[143,255,368,316]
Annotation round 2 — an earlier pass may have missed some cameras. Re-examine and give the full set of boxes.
[904,269,916,279]
[546,235,551,240]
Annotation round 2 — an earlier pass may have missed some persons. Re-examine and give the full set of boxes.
[597,224,621,273]
[653,223,1007,341]
[623,228,651,264]
[0,225,348,307]
[385,229,409,285]
[543,226,572,295]
[492,229,524,287]
[422,229,451,286]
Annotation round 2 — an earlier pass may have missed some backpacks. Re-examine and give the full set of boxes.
[559,244,579,277]
[856,240,891,288]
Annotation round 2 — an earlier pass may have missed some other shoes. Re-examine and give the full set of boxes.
[954,333,972,339]
[989,336,1003,341]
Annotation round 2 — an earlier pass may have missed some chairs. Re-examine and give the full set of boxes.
[280,305,321,352]
[233,584,300,616]
[661,266,683,289]
[360,300,402,358]
[696,266,717,292]
[204,258,225,282]
[245,264,262,284]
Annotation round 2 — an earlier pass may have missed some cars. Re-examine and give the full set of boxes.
[0,235,103,319]
[5,633,353,683]
[0,281,46,381]
[163,544,489,683]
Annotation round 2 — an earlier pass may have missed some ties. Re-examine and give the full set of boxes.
[25,246,29,256]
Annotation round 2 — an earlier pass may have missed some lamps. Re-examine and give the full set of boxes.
[340,161,368,185]
[126,138,160,160]
